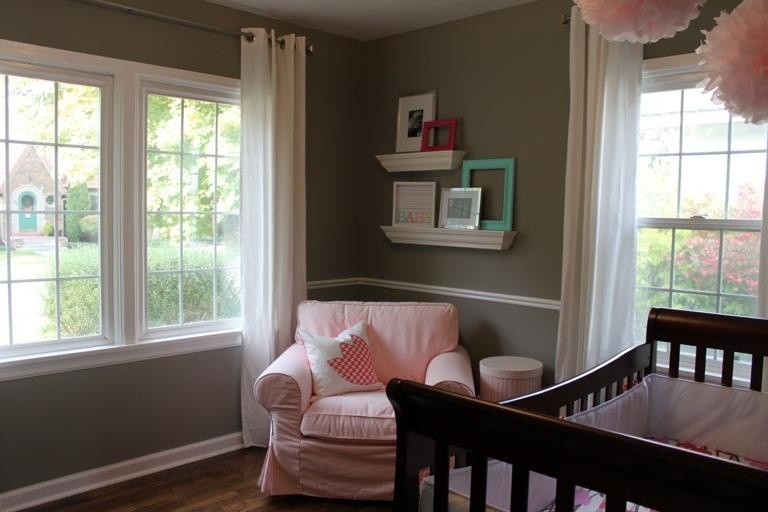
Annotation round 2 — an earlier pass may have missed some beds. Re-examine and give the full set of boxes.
[387,305,767,512]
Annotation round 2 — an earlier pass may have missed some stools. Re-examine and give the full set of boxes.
[479,355,543,404]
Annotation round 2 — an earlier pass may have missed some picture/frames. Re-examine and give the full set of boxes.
[461,159,514,231]
[437,187,481,230]
[394,90,438,152]
[393,181,436,230]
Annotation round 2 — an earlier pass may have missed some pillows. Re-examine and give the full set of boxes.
[297,318,386,400]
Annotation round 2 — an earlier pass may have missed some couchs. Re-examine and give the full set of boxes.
[250,299,476,501]
